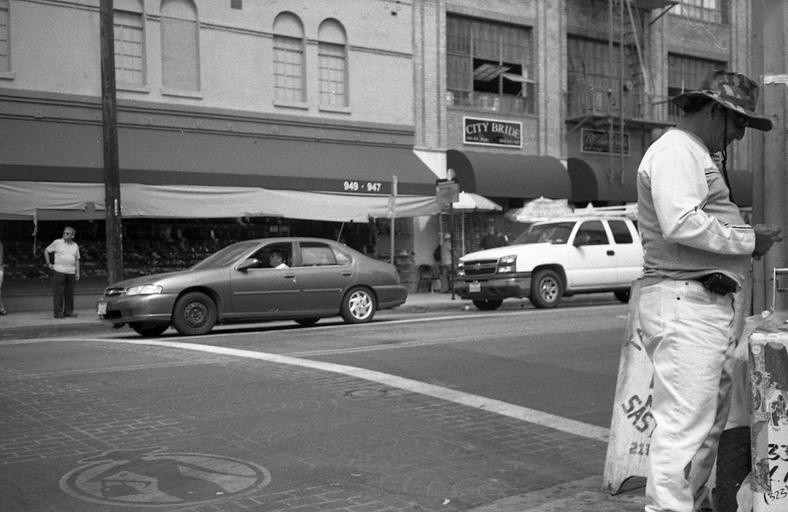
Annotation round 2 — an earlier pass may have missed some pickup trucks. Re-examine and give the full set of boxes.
[453,213,647,312]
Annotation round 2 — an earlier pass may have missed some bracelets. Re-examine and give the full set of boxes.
[47,262,52,267]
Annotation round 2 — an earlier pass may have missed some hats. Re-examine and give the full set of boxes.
[670,69,773,132]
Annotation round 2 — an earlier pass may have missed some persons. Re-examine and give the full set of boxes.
[0,241,8,315]
[633,70,784,512]
[439,233,451,293]
[267,250,291,271]
[479,225,507,249]
[44,226,82,320]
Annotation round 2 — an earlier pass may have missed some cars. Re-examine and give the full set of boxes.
[96,235,407,337]
